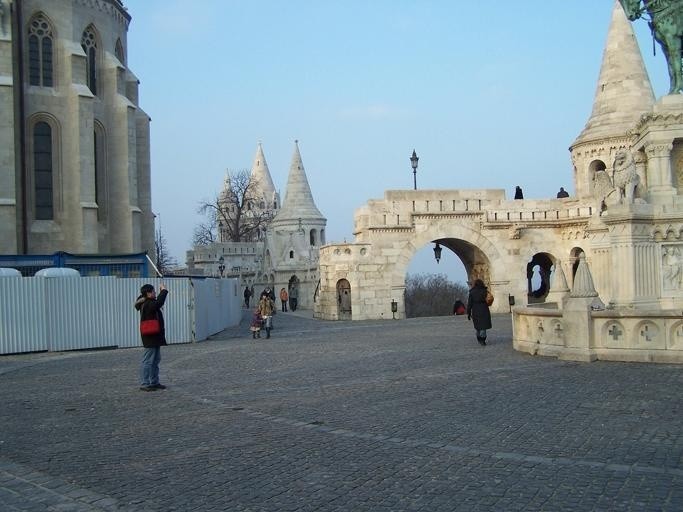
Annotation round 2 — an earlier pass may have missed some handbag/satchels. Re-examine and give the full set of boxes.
[456,307,464,314]
[485,291,494,306]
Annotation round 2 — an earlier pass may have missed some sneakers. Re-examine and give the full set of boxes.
[140,383,166,392]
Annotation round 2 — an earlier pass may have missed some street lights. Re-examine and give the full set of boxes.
[217,255,226,279]
[156,212,165,274]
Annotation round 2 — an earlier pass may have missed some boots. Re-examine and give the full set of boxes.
[477,336,487,346]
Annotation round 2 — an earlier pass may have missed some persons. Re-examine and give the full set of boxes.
[134,283,168,392]
[557,187,569,198]
[244,286,250,309]
[279,286,298,312]
[452,298,466,316]
[467,279,492,347]
[249,287,275,339]
[515,186,523,199]
